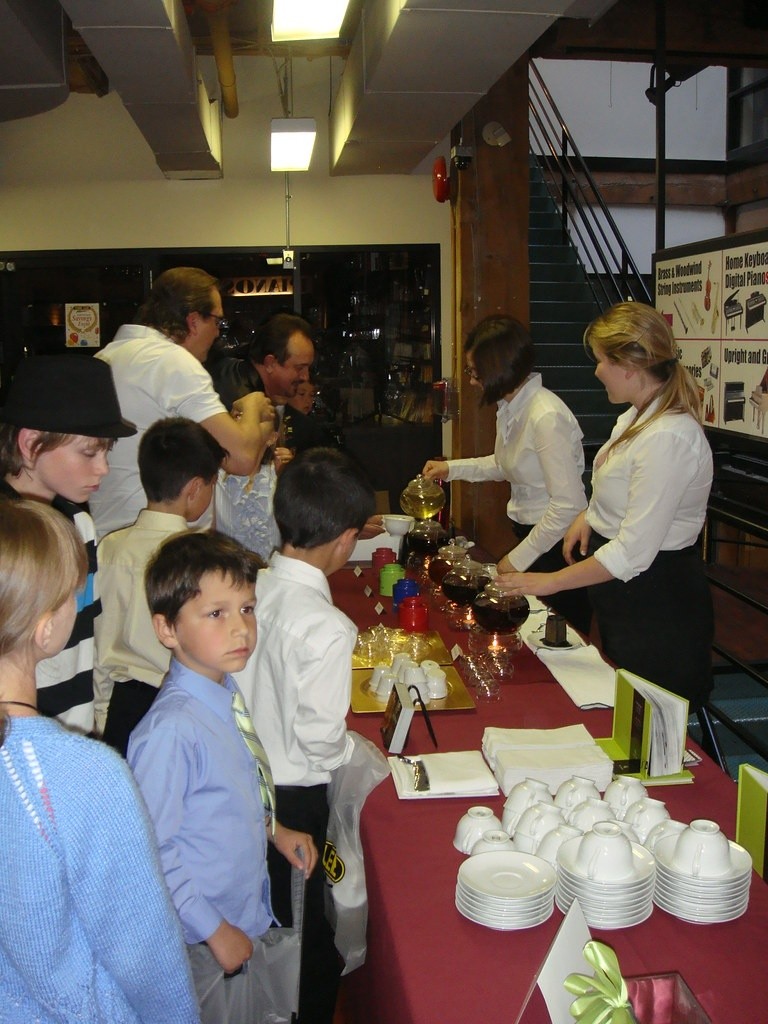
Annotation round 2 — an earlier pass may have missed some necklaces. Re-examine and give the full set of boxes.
[0,701,40,712]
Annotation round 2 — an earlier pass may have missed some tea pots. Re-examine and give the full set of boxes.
[405,519,448,556]
[472,581,530,634]
[400,474,446,518]
[429,540,468,584]
[441,554,492,605]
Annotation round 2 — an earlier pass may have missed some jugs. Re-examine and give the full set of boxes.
[354,626,432,664]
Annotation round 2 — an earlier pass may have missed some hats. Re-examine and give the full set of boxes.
[0,352,137,439]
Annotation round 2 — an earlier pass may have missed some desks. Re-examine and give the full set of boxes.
[328,558,768,1024]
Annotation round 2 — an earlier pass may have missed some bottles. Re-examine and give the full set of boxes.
[286,427,297,463]
[379,564,405,597]
[397,597,428,633]
[393,578,419,613]
[372,548,397,574]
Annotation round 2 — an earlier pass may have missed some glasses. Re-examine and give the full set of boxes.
[464,366,482,382]
[199,311,231,336]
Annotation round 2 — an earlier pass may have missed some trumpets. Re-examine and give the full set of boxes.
[690,301,705,328]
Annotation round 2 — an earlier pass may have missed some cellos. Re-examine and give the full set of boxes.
[704,260,712,311]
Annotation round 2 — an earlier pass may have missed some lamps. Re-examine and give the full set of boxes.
[270,46,316,171]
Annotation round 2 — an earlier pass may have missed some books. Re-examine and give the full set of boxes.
[735,763,768,882]
[595,669,695,786]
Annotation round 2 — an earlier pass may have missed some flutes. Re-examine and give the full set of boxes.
[672,300,689,335]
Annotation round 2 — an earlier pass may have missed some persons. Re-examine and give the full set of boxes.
[493,302,715,704]
[0,353,136,740]
[128,532,319,1024]
[0,502,204,1024]
[422,313,589,639]
[90,417,229,757]
[85,268,337,567]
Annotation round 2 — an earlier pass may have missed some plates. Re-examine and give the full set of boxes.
[653,833,752,924]
[387,750,500,799]
[527,632,580,650]
[555,835,655,929]
[455,850,556,930]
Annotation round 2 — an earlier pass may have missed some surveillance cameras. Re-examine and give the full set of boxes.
[451,145,474,170]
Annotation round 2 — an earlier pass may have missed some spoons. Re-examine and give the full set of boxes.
[531,623,544,633]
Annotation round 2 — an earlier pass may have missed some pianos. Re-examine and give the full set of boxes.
[746,291,767,332]
[724,289,743,336]
[723,381,746,425]
[748,365,768,434]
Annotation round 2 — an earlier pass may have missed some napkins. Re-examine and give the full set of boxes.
[481,724,614,798]
[516,593,616,710]
[387,750,500,800]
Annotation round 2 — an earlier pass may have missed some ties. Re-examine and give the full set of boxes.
[232,692,279,837]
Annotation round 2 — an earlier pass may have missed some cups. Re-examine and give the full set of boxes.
[545,615,567,642]
[373,515,412,538]
[369,653,448,704]
[673,819,732,879]
[481,563,496,576]
[332,884,367,952]
[452,774,689,880]
[459,650,514,703]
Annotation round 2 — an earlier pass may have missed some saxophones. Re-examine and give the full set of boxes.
[710,281,721,334]
